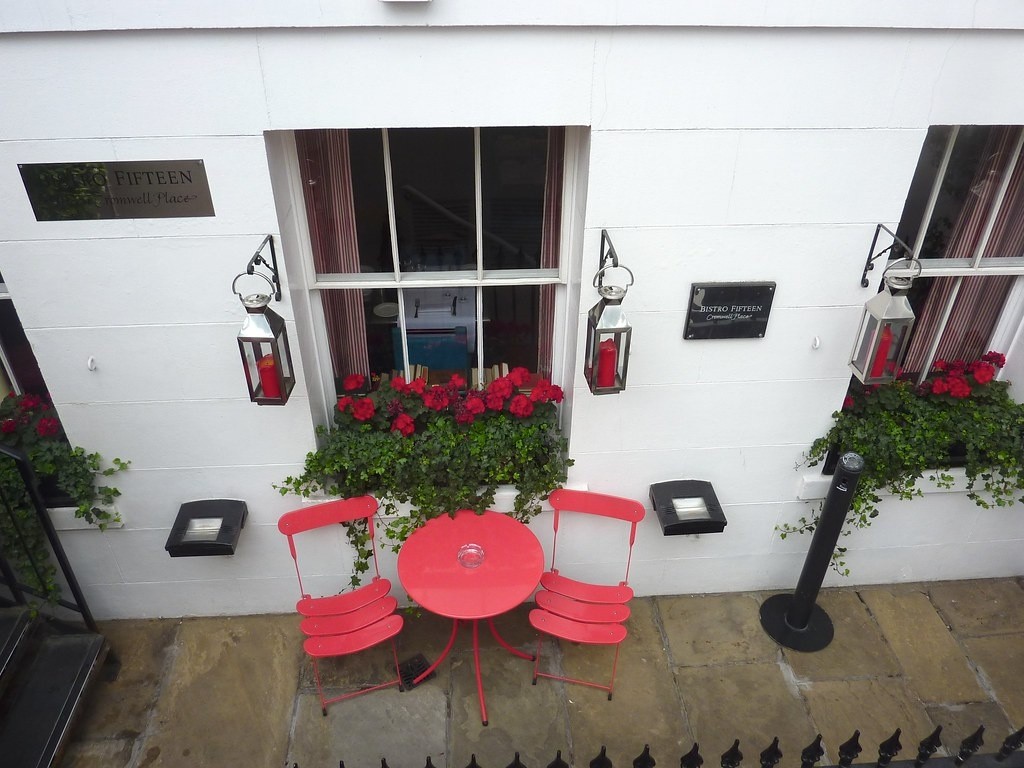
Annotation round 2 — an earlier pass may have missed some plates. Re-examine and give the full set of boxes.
[373,302,398,317]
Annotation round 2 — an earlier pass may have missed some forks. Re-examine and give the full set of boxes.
[414,298,420,318]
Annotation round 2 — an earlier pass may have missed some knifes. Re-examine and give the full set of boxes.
[452,296,458,317]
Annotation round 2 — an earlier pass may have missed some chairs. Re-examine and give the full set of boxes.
[278,494,403,716]
[529,488,645,700]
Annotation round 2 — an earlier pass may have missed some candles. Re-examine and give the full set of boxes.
[256,355,282,397]
[596,338,617,387]
[862,322,893,378]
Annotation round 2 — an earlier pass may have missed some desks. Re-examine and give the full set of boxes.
[398,508,544,726]
[363,288,398,375]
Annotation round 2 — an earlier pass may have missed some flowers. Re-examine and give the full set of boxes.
[271,367,575,618]
[768,347,1024,575]
[0,389,130,620]
